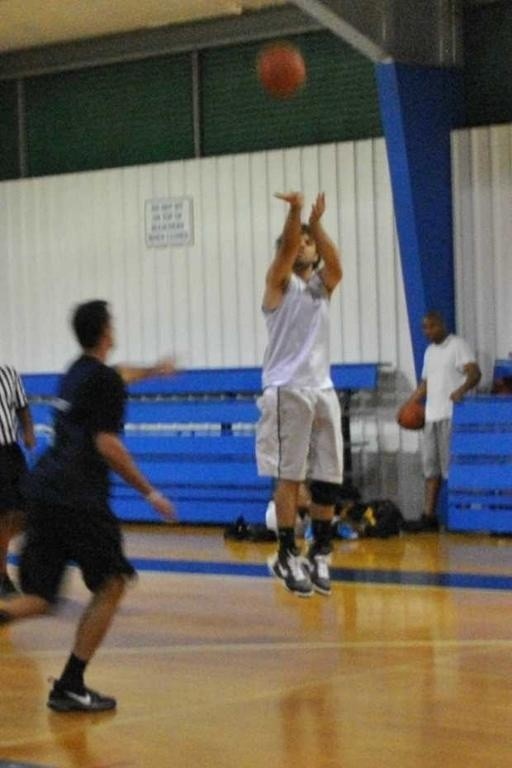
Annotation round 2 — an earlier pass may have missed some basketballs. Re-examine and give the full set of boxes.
[259,45,305,94]
[398,403,425,430]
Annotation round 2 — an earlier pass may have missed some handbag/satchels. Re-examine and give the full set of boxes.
[346,499,404,538]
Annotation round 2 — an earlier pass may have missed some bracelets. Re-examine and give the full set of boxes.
[146,490,160,504]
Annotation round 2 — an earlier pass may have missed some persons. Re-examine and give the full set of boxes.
[1,298,175,712]
[254,192,344,599]
[1,364,34,599]
[405,311,482,534]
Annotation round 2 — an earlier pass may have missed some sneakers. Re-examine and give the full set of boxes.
[46,679,116,712]
[400,512,441,532]
[271,550,314,597]
[310,547,332,595]
